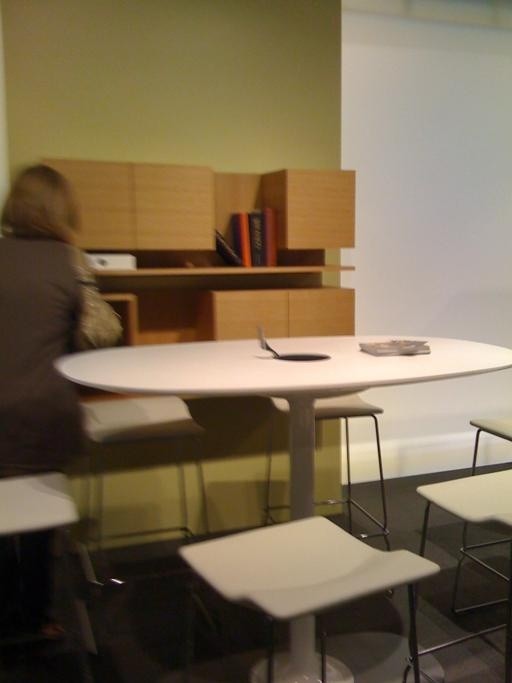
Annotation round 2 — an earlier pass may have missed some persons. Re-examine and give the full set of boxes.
[0,163,126,635]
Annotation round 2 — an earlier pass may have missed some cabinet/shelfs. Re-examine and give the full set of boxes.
[37,157,357,402]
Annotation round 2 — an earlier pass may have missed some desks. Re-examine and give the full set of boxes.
[53,334,512,682]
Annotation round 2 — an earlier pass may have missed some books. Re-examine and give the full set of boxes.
[215,229,241,266]
[232,211,252,267]
[261,207,278,267]
[248,211,264,267]
[360,339,432,357]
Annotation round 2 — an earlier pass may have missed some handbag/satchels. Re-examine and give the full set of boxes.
[68,246,124,352]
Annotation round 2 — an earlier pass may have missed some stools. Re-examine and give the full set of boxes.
[1,393,512,683]
